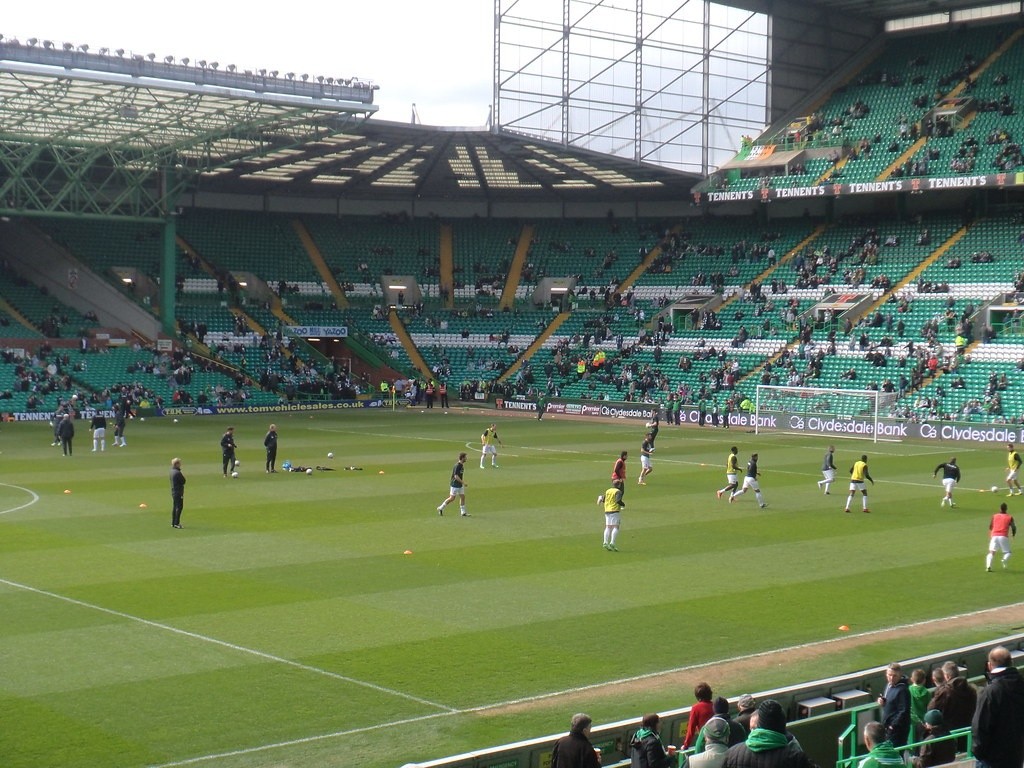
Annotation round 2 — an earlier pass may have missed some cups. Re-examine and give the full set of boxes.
[879,693,886,702]
[594,747,601,758]
[668,745,676,757]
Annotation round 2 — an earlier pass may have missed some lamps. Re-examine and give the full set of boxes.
[133,52,157,64]
[259,70,278,79]
[245,68,254,76]
[64,41,90,53]
[0,34,54,49]
[318,76,380,92]
[163,55,190,67]
[195,59,219,69]
[286,73,310,82]
[224,62,238,73]
[98,48,125,57]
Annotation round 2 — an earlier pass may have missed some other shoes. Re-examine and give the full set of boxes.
[950,503,956,507]
[610,542,618,552]
[824,492,830,494]
[863,509,870,512]
[716,490,722,499]
[597,495,603,506]
[845,508,850,513]
[987,567,991,572]
[461,513,471,517]
[638,482,647,486]
[1007,493,1013,497]
[761,504,767,508]
[603,543,612,551]
[171,524,184,528]
[942,498,946,507]
[818,480,822,489]
[729,497,733,504]
[1002,559,1008,570]
[1015,493,1023,495]
[437,507,443,516]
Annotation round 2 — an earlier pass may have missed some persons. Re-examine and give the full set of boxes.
[637,432,653,486]
[50,404,75,457]
[169,457,186,529]
[860,646,1024,768]
[648,409,659,453]
[550,682,814,768]
[844,455,875,513]
[984,502,1016,571]
[264,423,279,473]
[480,424,503,469]
[716,52,1024,191]
[112,404,126,447]
[1003,443,1023,497]
[603,479,625,552]
[597,451,628,509]
[816,446,836,495]
[436,451,471,518]
[454,224,1024,428]
[220,426,237,478]
[88,410,106,452]
[0,230,453,419]
[932,457,960,507]
[716,447,742,501]
[729,453,769,508]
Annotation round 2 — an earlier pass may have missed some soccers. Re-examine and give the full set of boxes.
[174,419,178,423]
[130,416,134,420]
[328,453,333,458]
[232,472,239,478]
[235,460,240,466]
[991,486,998,493]
[140,418,144,421]
[306,468,313,475]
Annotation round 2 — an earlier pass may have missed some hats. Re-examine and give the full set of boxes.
[740,693,755,708]
[713,696,729,715]
[758,699,786,735]
[924,709,943,726]
[704,717,731,741]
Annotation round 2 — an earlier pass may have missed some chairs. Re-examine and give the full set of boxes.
[683,19,1024,192]
[0,199,1024,421]
